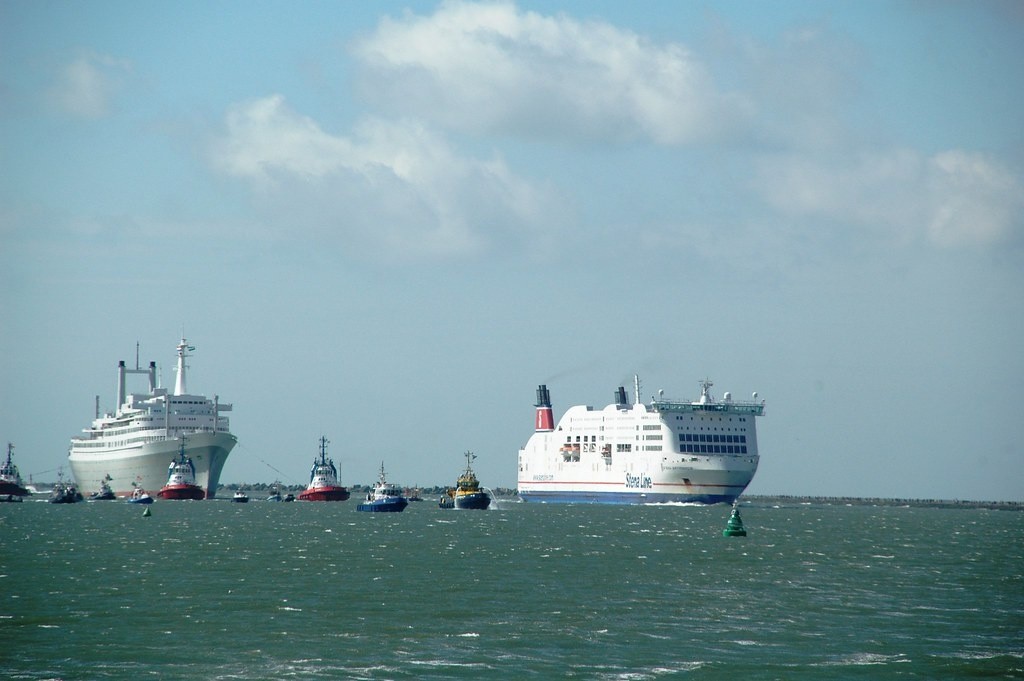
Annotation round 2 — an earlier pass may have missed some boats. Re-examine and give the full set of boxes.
[155,433,206,501]
[355,459,409,513]
[232,486,250,503]
[126,483,154,503]
[0,442,33,503]
[283,493,295,503]
[48,471,84,504]
[437,449,492,511]
[266,483,284,502]
[408,484,423,502]
[294,434,351,502]
[86,478,116,500]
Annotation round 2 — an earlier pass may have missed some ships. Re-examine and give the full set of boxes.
[514,372,766,505]
[65,322,240,500]
